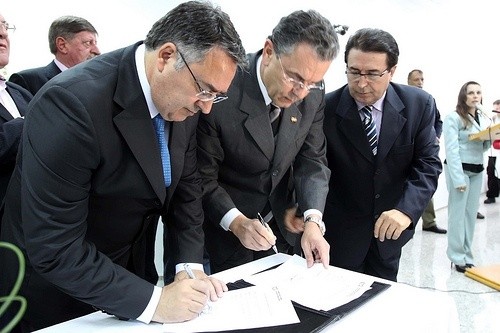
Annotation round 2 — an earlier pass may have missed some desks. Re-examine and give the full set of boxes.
[32,253,430,333]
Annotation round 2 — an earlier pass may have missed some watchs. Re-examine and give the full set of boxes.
[304,216,327,237]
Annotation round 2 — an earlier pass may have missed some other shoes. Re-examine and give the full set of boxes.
[477,212,485,219]
[422,225,447,234]
[484,197,495,203]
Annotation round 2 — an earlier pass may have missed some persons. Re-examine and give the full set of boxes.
[8,15,102,98]
[321,26,441,283]
[197,9,339,274]
[443,81,500,273]
[408,69,447,235]
[0,13,34,197]
[0,0,252,333]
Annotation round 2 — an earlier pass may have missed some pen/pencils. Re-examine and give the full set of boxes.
[256,211,279,255]
[183,263,197,280]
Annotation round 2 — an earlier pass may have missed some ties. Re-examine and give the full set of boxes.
[359,105,378,162]
[153,113,171,188]
[269,102,282,151]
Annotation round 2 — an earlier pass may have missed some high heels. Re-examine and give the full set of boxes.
[451,261,475,273]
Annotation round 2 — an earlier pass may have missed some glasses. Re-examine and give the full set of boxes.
[344,64,391,82]
[177,50,228,104]
[0,22,16,33]
[277,53,325,92]
